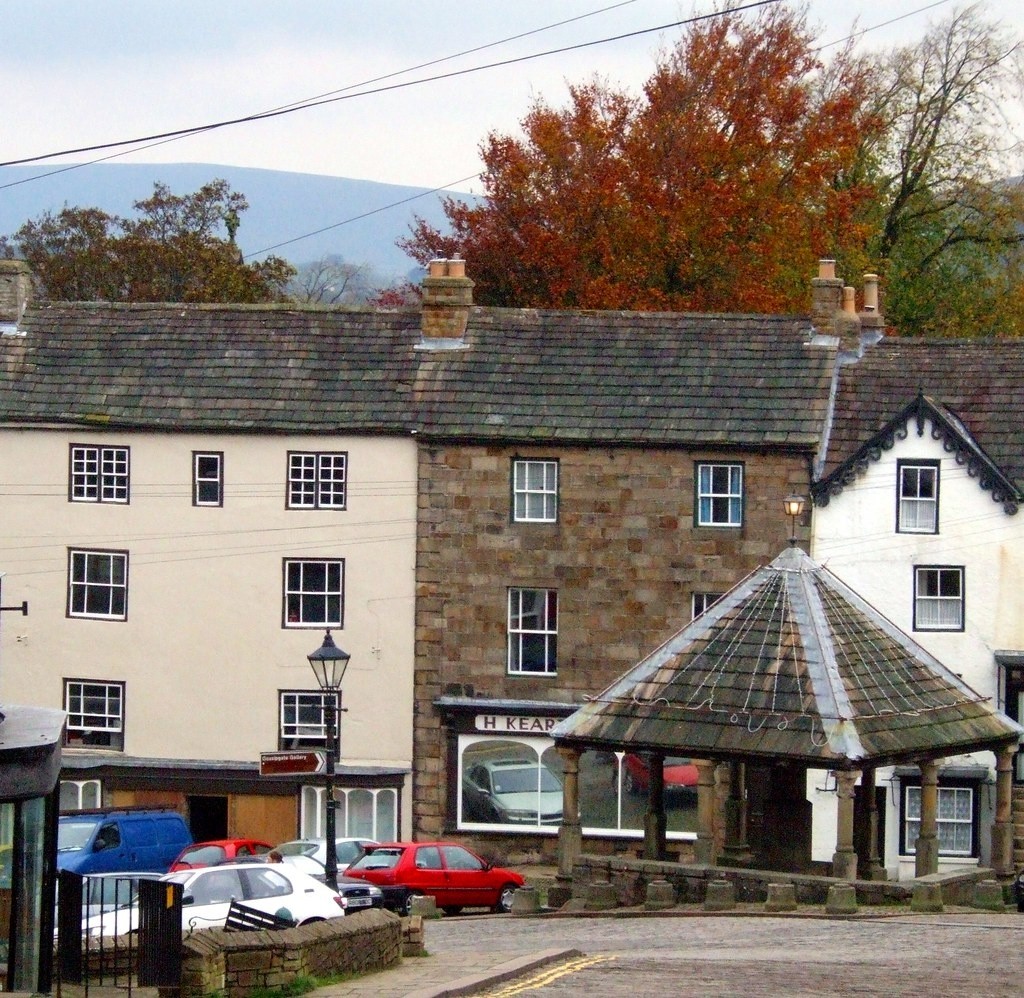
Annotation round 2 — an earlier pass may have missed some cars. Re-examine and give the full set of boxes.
[344,841,526,913]
[269,838,378,882]
[612,752,698,804]
[170,840,274,874]
[52,873,164,928]
[52,864,348,940]
[464,759,582,827]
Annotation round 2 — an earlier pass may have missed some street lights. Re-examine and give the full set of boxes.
[306,627,352,895]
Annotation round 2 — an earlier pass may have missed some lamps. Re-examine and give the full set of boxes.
[782,488,806,548]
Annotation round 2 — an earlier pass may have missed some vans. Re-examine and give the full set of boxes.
[55,804,193,880]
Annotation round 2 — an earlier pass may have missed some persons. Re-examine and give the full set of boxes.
[266,851,283,863]
